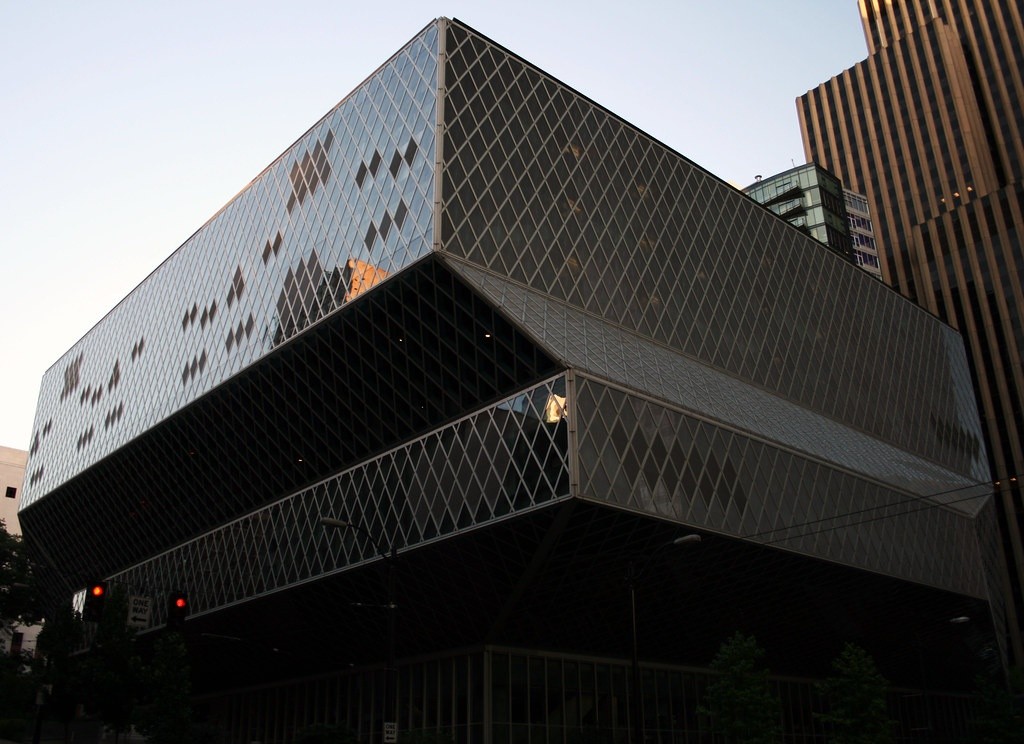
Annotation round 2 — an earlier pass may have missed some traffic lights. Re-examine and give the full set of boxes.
[166,592,187,634]
[82,577,109,624]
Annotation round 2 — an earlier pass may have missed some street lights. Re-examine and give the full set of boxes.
[318,516,398,744]
[628,533,703,744]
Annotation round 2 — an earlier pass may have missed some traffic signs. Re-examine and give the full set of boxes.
[125,595,153,627]
[383,722,397,744]
